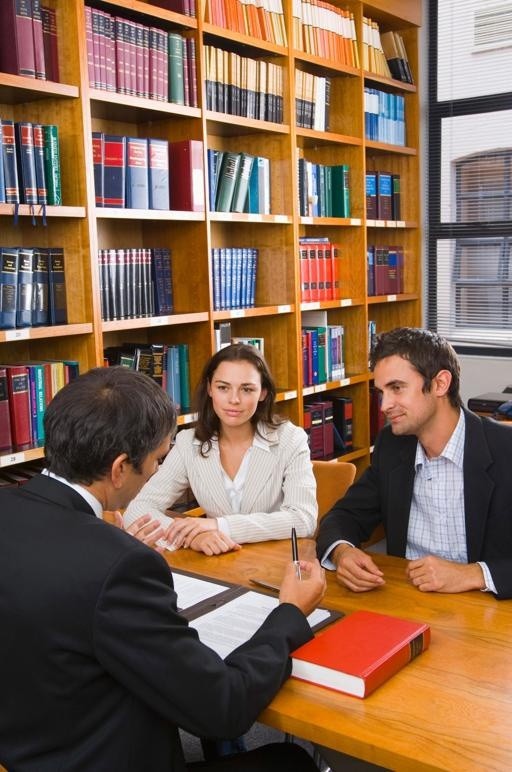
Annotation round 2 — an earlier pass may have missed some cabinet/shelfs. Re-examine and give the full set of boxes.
[0,0,424,552]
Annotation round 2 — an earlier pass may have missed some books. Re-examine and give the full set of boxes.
[167,566,345,663]
[0,1,78,480]
[85,0,204,413]
[203,0,288,352]
[293,1,354,460]
[360,17,411,444]
[285,608,431,700]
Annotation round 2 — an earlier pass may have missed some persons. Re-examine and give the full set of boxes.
[1,362,331,770]
[312,324,512,602]
[114,339,321,559]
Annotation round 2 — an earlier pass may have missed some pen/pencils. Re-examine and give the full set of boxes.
[249,579,280,593]
[292,523,301,580]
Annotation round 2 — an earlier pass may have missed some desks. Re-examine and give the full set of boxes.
[96,506,512,772]
